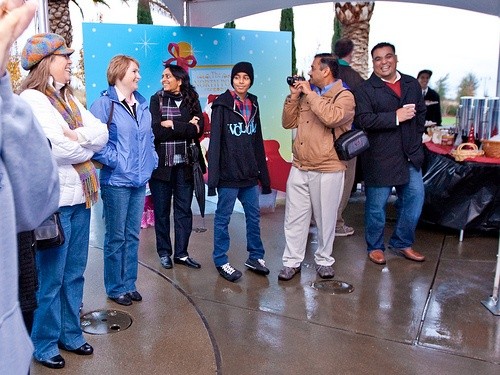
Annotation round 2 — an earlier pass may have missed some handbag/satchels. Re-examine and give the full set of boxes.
[32,212,65,251]
[331,88,370,161]
[90,94,114,168]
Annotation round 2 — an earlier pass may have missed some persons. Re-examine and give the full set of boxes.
[416,70,442,128]
[17,32,109,369]
[0,0,60,375]
[149,64,207,269]
[207,62,272,282]
[88,55,158,305]
[354,42,427,264]
[278,53,356,281]
[305,38,366,236]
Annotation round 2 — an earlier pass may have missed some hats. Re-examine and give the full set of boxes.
[21,33,75,70]
[231,62,254,89]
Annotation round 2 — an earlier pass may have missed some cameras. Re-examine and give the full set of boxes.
[287,76,305,87]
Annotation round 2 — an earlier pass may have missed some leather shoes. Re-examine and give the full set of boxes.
[369,249,386,264]
[108,291,142,306]
[173,255,201,269]
[397,248,425,261]
[160,256,173,268]
[40,354,65,368]
[58,342,94,356]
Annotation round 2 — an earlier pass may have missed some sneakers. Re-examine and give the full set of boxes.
[334,223,355,237]
[315,265,335,278]
[216,262,242,281]
[278,263,302,281]
[244,258,270,275]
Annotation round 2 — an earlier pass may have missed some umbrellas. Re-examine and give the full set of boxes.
[187,131,204,219]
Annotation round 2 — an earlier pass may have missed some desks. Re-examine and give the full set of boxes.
[422,142,500,242]
